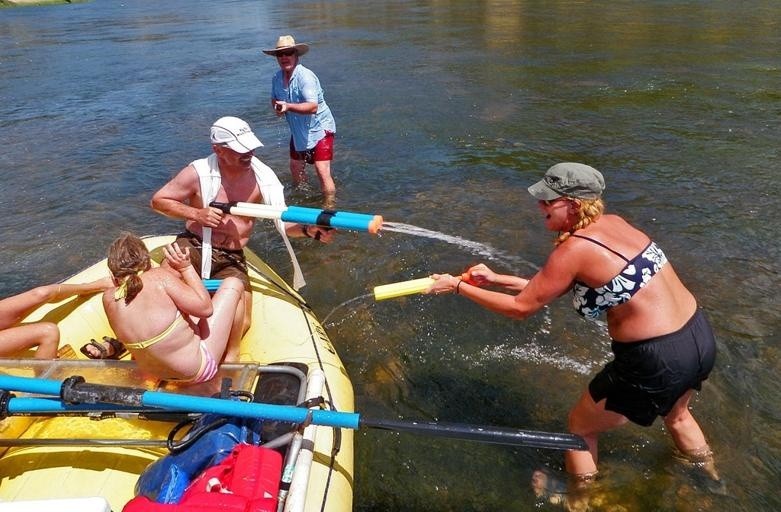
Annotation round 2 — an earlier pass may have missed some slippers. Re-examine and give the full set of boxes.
[535,476,569,508]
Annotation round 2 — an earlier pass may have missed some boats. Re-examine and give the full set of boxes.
[0,232,354,511]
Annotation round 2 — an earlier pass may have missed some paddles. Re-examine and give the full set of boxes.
[1,373,591,450]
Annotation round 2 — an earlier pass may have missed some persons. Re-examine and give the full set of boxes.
[80,115,340,355]
[425,162,728,512]
[262,33,336,191]
[100,234,246,393]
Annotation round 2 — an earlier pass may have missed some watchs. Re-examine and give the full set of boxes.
[0,278,113,361]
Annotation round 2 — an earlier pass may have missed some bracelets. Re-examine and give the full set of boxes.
[303,224,313,238]
[455,279,464,295]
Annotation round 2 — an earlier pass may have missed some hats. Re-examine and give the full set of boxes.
[210,115,265,155]
[262,35,310,57]
[527,162,605,202]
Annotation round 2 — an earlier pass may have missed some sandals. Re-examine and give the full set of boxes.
[80,336,126,358]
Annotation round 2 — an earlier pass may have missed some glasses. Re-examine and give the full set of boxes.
[274,49,296,56]
